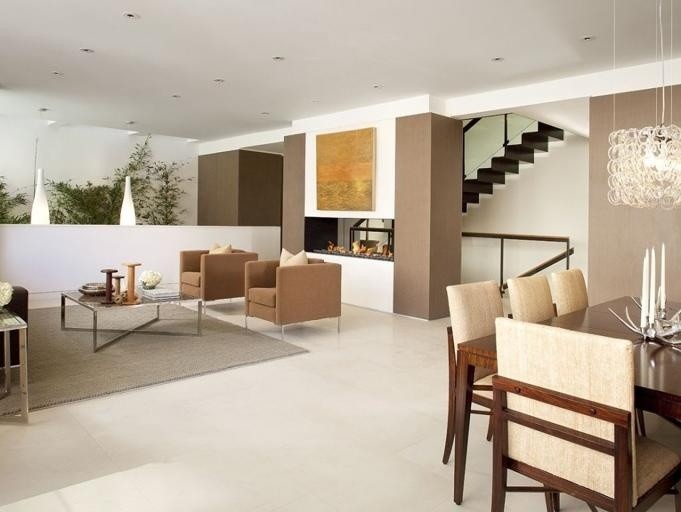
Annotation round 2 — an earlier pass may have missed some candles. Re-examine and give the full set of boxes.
[648,246,656,324]
[640,247,650,326]
[660,243,666,310]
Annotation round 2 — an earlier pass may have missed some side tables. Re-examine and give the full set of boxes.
[0,309,29,424]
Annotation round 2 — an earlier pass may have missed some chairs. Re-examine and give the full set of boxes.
[506,273,556,323]
[179,247,258,301]
[442,280,560,512]
[0,286,29,368]
[490,315,681,512]
[244,258,342,336]
[552,267,590,317]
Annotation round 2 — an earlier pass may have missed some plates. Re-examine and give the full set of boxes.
[81,282,113,289]
[77,288,114,295]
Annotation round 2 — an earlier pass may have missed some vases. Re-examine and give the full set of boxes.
[119,176,136,226]
[30,168,51,225]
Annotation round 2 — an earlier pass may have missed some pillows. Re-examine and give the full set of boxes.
[208,241,233,255]
[279,247,309,265]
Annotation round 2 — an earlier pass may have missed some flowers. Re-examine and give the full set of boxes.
[0,280,14,307]
[138,271,162,288]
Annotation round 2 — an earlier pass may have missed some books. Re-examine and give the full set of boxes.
[137,285,181,302]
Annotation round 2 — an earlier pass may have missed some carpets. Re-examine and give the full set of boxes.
[0,300,311,417]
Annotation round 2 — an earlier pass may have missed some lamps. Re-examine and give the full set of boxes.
[606,0,681,209]
